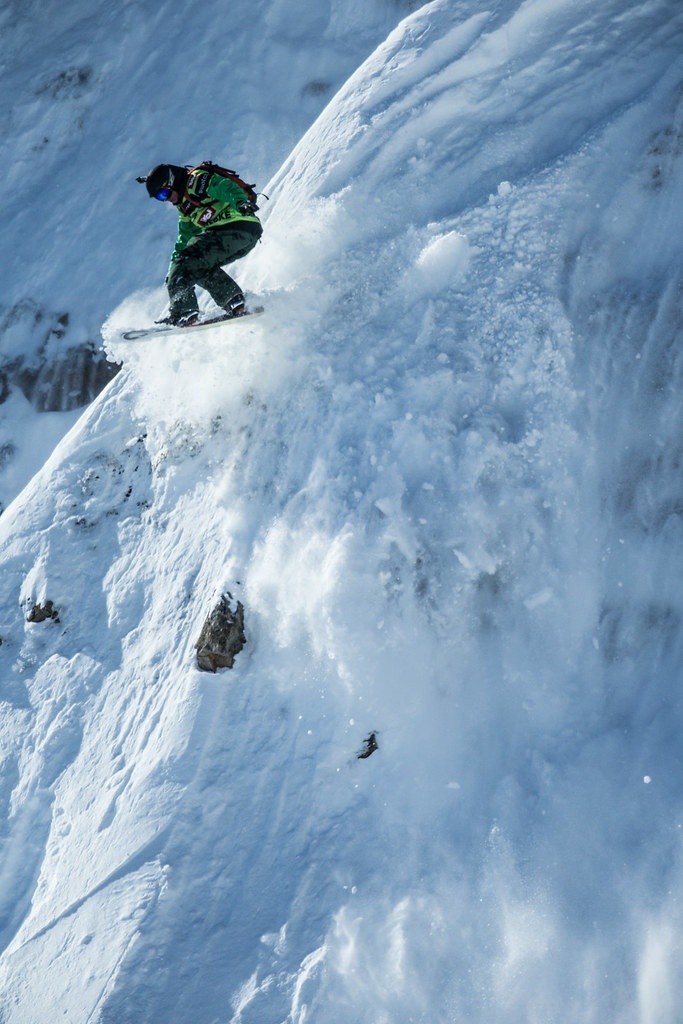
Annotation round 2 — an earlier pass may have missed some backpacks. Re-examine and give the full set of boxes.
[184,160,257,208]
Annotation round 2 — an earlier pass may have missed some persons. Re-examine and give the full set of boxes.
[146,164,265,327]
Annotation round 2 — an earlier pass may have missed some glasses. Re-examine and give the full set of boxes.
[155,189,170,202]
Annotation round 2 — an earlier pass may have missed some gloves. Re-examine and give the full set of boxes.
[238,199,260,217]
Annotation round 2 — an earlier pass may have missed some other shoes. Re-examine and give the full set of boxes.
[171,311,198,325]
[223,294,245,311]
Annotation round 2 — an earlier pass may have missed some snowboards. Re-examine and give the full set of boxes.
[110,305,271,343]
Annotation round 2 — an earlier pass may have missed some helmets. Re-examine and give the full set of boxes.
[146,163,188,205]
